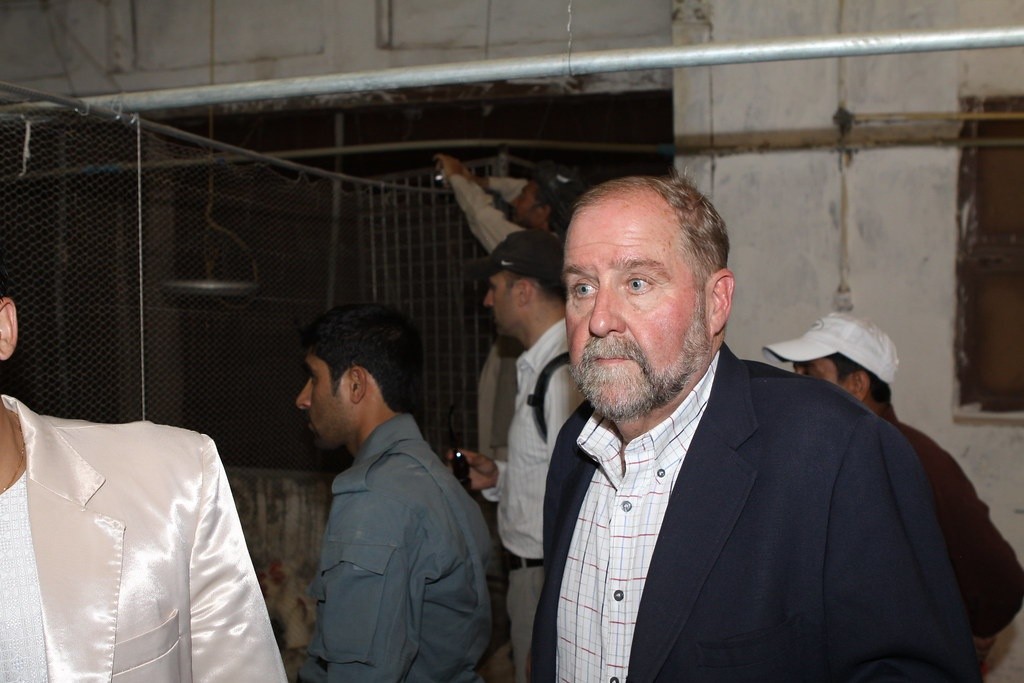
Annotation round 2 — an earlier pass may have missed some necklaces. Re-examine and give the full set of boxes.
[4,407,25,492]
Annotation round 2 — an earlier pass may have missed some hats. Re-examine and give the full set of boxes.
[459,229,563,285]
[763,311,900,384]
[526,158,586,209]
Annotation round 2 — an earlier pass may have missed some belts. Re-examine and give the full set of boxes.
[502,551,543,570]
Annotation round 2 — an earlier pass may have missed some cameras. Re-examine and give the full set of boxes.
[435,168,449,186]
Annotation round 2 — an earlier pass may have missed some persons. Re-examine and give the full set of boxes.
[0,271,289,683]
[294,305,494,683]
[525,174,986,683]
[435,144,1024,682]
